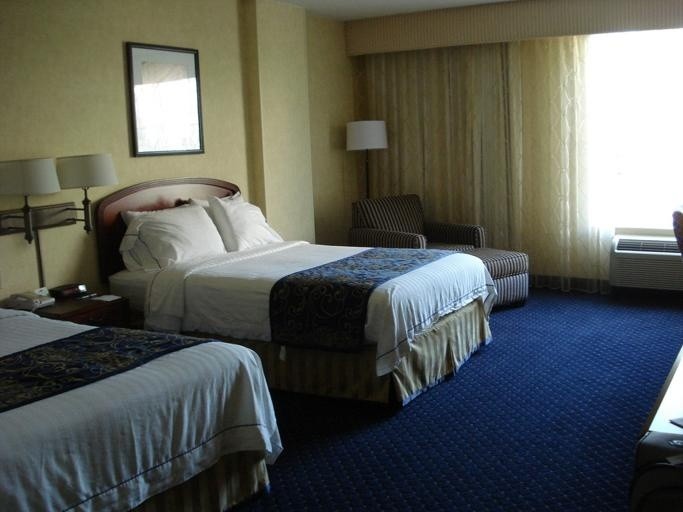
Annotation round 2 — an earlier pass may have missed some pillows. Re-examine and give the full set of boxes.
[115,189,284,274]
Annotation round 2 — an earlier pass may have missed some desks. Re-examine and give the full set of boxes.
[625,340,682,512]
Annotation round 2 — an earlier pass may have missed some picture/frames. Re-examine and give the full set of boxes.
[121,38,206,159]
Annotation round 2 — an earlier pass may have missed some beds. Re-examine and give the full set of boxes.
[88,175,499,412]
[0,308,284,511]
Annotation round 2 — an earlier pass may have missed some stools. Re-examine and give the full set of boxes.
[458,245,529,311]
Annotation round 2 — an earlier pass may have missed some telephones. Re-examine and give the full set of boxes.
[1,291,56,313]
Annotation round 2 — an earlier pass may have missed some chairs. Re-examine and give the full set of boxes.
[344,190,484,254]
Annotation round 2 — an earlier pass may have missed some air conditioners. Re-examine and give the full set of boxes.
[605,231,683,295]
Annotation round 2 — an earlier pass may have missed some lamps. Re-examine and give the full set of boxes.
[342,119,390,200]
[0,152,118,245]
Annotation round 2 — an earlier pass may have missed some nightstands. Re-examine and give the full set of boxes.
[0,281,133,330]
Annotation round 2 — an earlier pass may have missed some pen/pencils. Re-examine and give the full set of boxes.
[80,294,96,300]
